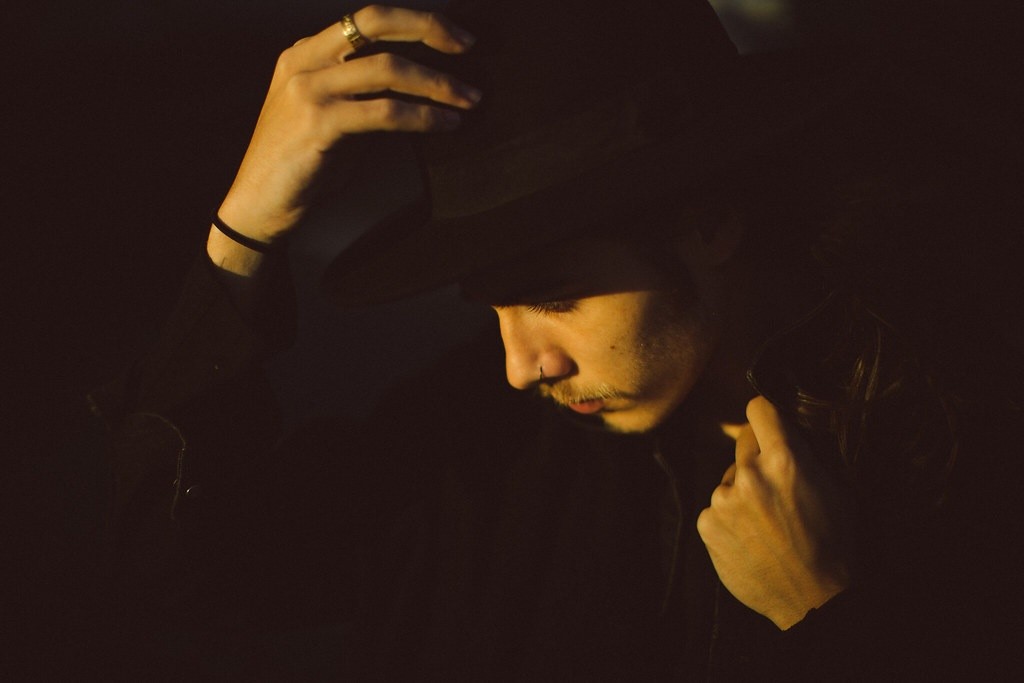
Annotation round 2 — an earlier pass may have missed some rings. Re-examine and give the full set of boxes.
[342,12,367,51]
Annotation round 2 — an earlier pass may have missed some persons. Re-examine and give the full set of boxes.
[83,0,958,683]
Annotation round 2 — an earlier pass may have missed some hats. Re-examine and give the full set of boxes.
[311,2,846,305]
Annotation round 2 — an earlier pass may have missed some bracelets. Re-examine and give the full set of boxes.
[210,208,274,254]
